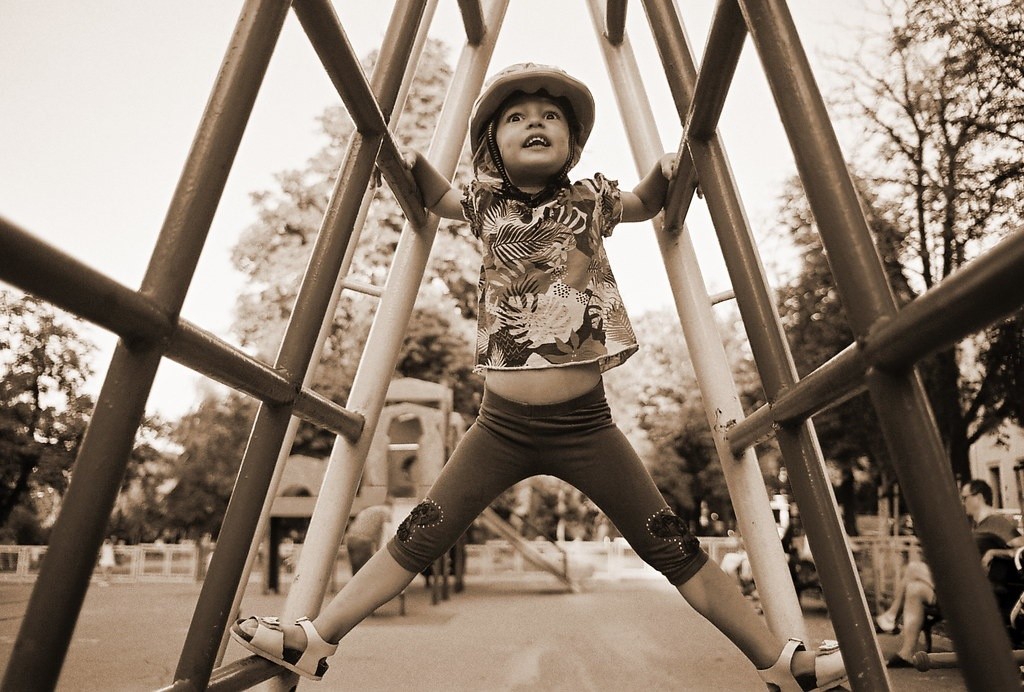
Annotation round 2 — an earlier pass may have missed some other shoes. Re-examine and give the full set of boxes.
[885,653,914,669]
[872,617,900,636]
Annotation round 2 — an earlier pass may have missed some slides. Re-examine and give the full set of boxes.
[478,497,581,591]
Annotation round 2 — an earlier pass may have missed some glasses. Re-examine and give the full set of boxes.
[961,493,972,502]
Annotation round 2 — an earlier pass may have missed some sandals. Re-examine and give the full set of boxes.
[229,614,339,682]
[757,637,851,692]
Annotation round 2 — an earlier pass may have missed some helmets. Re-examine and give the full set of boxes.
[468,62,596,179]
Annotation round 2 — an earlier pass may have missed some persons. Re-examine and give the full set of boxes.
[345,495,396,577]
[97,539,117,588]
[719,479,1024,671]
[228,64,850,692]
